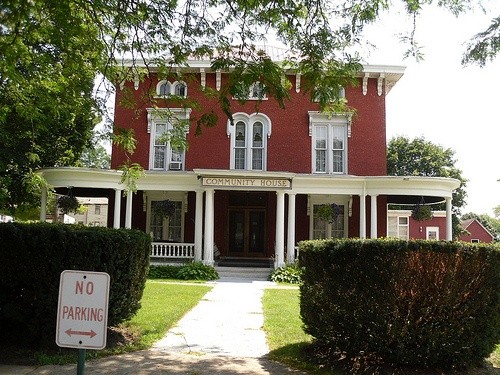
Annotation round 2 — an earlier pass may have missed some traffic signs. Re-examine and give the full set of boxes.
[55,269,110,350]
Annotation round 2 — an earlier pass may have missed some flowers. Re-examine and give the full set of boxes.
[318,202,340,220]
[157,200,173,216]
[56,196,80,215]
[410,203,433,222]
